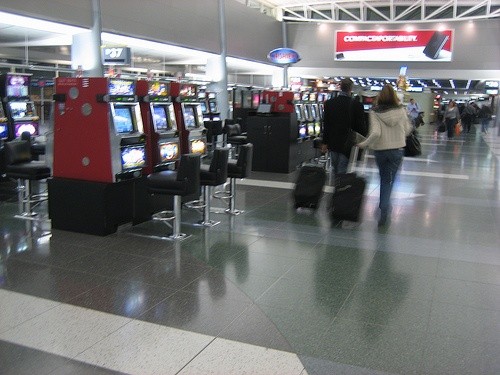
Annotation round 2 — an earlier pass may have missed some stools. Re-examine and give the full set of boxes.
[7,141,51,216]
[148,118,253,241]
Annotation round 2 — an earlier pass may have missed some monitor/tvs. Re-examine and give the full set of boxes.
[6,74,30,97]
[199,101,208,113]
[7,101,37,120]
[190,139,206,157]
[183,103,198,128]
[209,101,218,113]
[158,141,180,163]
[119,144,147,172]
[112,103,138,135]
[12,121,40,137]
[151,103,171,132]
[292,104,324,139]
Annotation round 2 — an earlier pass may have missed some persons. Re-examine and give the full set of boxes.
[408,97,491,137]
[319,78,364,226]
[20,130,37,164]
[357,83,414,227]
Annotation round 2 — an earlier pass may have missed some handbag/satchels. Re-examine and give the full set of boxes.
[403,134,422,157]
[456,122,463,136]
[438,122,446,132]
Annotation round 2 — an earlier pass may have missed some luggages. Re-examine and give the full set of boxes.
[294,151,329,211]
[330,144,370,228]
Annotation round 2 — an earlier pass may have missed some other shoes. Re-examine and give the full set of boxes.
[378,209,387,226]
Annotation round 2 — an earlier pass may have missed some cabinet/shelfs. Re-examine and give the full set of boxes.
[248,113,297,173]
